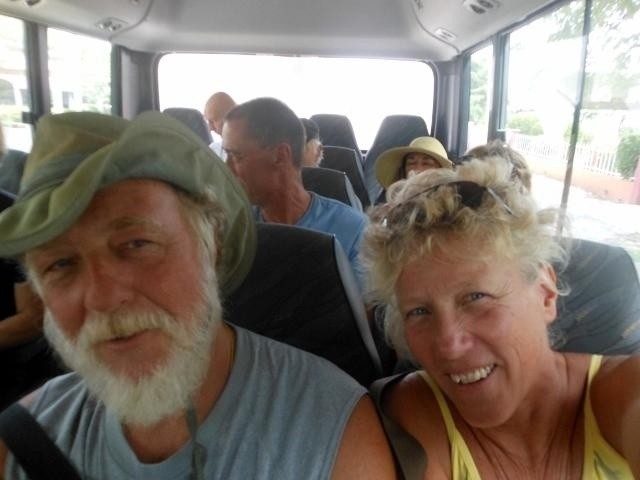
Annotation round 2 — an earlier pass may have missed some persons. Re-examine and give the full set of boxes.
[1,190,47,344]
[204,93,456,369]
[1,93,393,479]
[367,142,638,480]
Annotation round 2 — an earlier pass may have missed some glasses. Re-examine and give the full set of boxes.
[378,181,520,226]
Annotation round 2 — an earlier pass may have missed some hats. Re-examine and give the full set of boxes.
[1,109,257,297]
[375,136,453,191]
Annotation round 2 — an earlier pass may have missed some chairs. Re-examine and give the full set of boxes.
[1,103,429,380]
[542,225,638,357]
